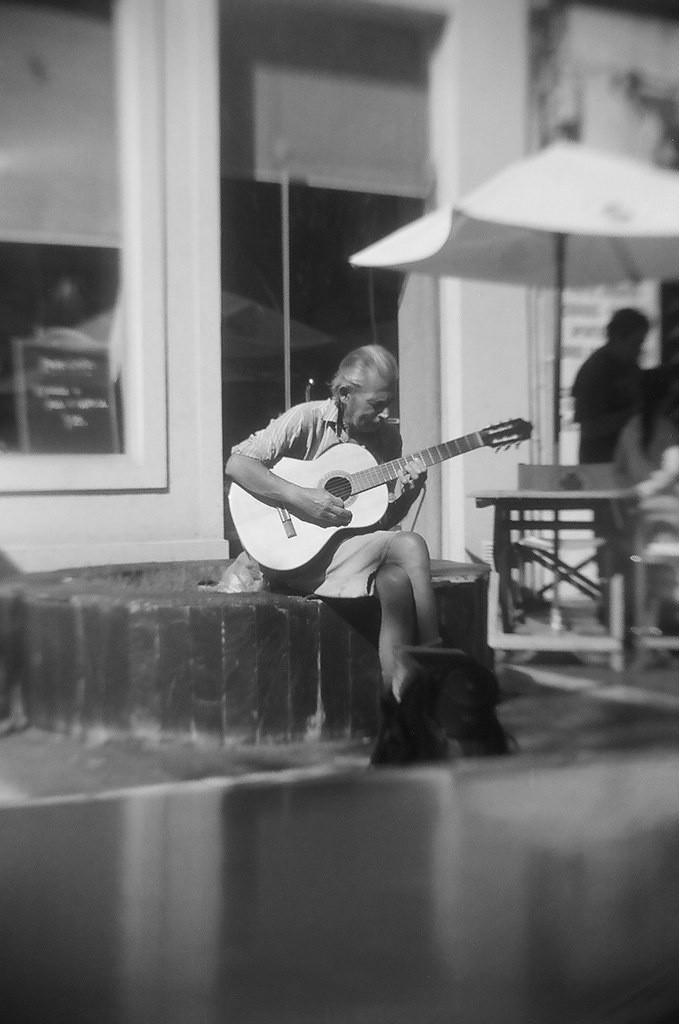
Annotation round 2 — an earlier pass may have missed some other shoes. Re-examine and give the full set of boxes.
[369,661,450,767]
[423,638,508,755]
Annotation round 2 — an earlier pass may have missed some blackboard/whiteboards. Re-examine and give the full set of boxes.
[11,335,118,456]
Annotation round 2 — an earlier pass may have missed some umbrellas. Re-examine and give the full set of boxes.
[348,138,679,629]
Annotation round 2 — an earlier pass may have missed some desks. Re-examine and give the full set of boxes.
[471,489,641,673]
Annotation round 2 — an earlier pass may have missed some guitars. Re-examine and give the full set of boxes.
[229,415,534,579]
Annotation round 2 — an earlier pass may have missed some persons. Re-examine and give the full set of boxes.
[225,344,438,685]
[573,307,662,630]
